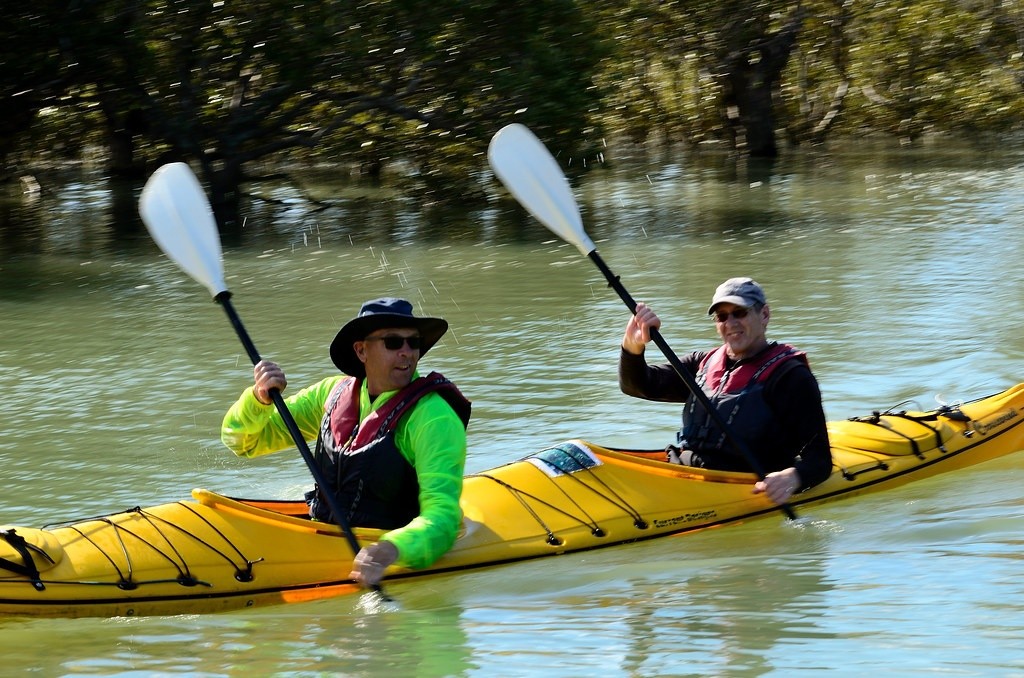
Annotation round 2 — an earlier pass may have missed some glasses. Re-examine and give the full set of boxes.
[714,303,752,322]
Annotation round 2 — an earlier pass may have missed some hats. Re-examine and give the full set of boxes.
[330,298,448,377]
[365,335,422,350]
[708,277,766,316]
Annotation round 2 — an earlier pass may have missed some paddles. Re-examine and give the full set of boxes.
[487,123,799,521]
[137,162,401,618]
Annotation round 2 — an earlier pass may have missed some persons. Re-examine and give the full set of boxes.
[618,276,833,508]
[222,297,466,584]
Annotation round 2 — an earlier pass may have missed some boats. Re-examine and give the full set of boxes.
[0,373,1023,602]
